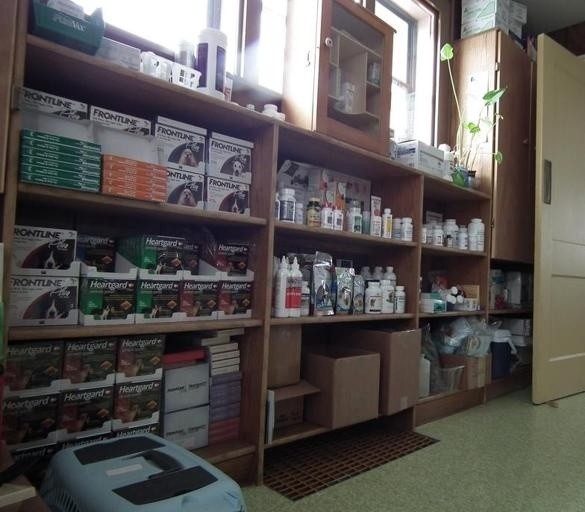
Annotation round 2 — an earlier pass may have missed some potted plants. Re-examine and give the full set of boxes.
[438,42,505,189]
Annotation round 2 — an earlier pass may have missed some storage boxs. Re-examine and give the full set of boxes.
[3,329,247,469]
[439,352,494,391]
[1,250,253,326]
[266,324,423,431]
[460,0,528,49]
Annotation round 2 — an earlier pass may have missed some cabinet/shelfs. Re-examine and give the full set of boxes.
[448,26,537,404]
[0,32,493,488]
[1,0,13,196]
[279,0,399,158]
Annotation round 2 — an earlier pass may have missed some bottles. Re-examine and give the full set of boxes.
[275,189,413,242]
[358,264,407,315]
[262,103,286,121]
[422,219,487,252]
[224,70,233,103]
[438,143,478,189]
[195,28,228,101]
[173,40,195,71]
[270,255,311,318]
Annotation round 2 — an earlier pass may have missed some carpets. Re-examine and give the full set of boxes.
[262,422,443,504]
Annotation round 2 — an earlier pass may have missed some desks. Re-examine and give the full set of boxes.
[0,435,51,511]
